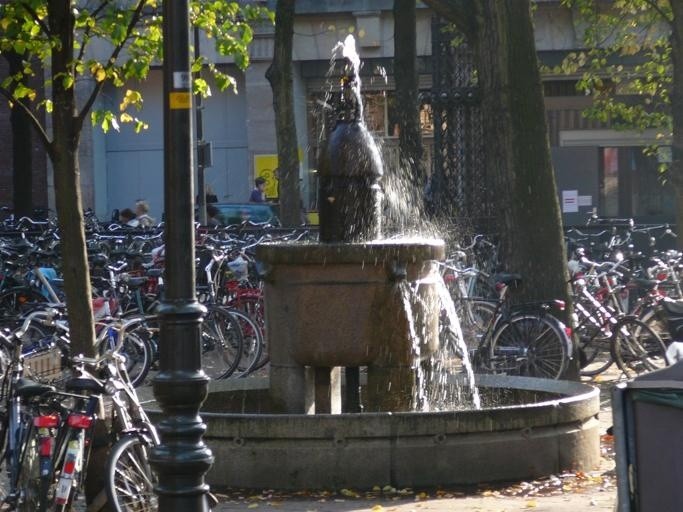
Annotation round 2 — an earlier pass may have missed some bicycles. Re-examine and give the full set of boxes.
[0,384,159,512]
[435,207,682,382]
[0,202,319,391]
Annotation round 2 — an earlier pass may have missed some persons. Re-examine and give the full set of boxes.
[196,183,218,202]
[206,204,222,227]
[136,199,156,228]
[248,178,273,204]
[119,208,142,228]
[273,168,280,204]
